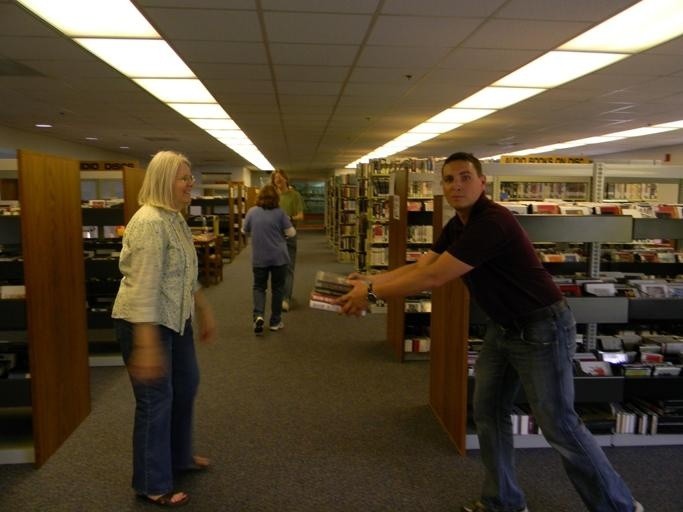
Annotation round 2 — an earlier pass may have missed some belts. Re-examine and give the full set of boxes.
[500,300,570,328]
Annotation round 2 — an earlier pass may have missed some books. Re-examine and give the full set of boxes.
[508,404,548,436]
[80,158,258,311]
[608,398,683,435]
[0,199,20,216]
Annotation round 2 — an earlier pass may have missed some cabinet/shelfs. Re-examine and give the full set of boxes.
[180,172,246,287]
[325,159,393,275]
[2,147,90,473]
[428,159,683,457]
[79,165,147,368]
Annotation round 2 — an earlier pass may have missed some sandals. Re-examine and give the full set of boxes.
[136,490,190,507]
[179,456,210,471]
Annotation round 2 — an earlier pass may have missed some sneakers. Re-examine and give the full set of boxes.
[254,317,264,334]
[282,300,291,313]
[461,499,528,512]
[270,321,284,330]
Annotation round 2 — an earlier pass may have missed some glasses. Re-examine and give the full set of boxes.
[175,175,197,183]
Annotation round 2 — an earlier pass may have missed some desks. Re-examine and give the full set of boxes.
[387,160,434,363]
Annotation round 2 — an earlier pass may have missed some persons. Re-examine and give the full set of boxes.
[330,152,644,512]
[241,169,304,334]
[111,150,214,507]
